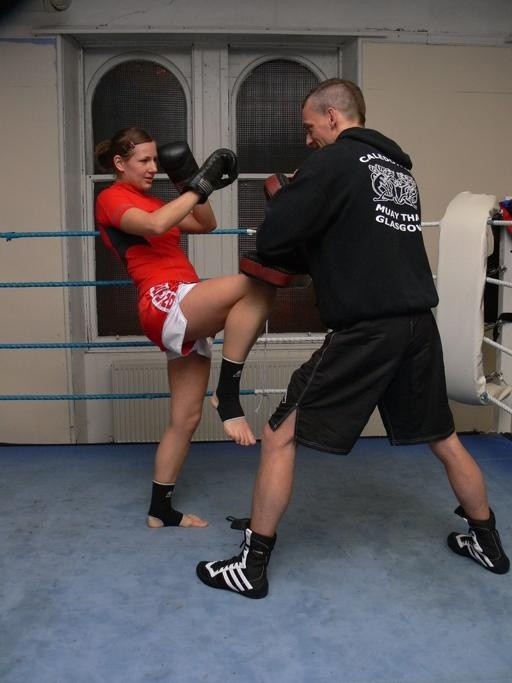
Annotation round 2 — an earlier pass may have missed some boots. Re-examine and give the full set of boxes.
[195,528,278,600]
[446,505,511,575]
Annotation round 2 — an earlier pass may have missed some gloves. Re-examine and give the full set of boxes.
[157,141,199,195]
[181,148,239,204]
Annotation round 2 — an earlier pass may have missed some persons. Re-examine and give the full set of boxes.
[194,77,510,597]
[95,126,277,528]
